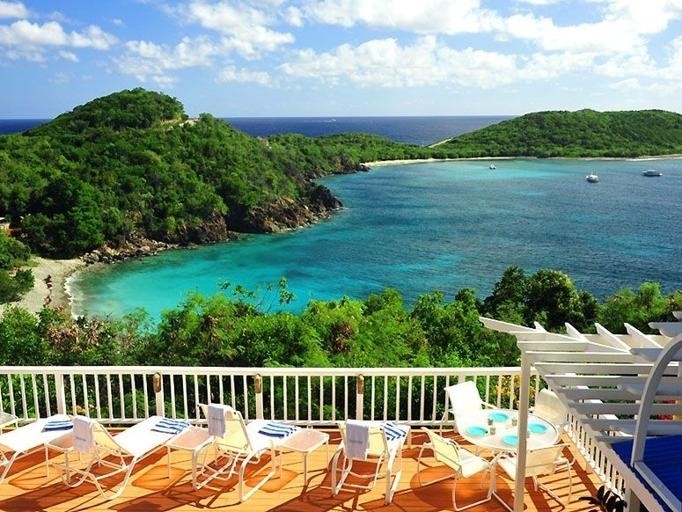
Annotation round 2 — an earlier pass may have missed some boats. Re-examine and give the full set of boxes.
[489,164,496,169]
[642,169,663,176]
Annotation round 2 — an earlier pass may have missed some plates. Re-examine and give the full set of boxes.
[466,426,488,436]
[528,423,547,433]
[487,412,510,423]
[502,435,518,445]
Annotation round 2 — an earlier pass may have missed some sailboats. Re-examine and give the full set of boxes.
[586,168,599,183]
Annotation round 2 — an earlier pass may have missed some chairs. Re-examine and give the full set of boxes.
[438,381,501,458]
[532,387,578,477]
[46,413,192,502]
[416,426,494,512]
[491,442,573,512]
[189,403,300,503]
[329,419,413,503]
[1,411,77,480]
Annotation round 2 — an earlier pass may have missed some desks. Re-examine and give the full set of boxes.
[457,408,562,490]
[165,425,215,486]
[45,431,77,484]
[276,426,331,489]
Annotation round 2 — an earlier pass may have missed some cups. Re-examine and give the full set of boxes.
[488,416,496,435]
[512,415,517,426]
